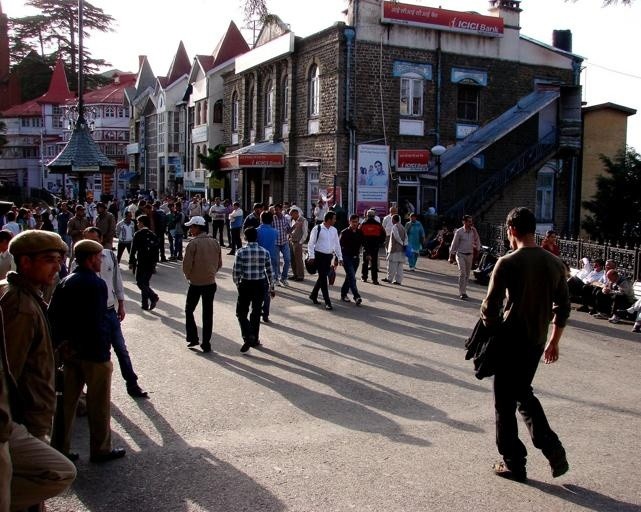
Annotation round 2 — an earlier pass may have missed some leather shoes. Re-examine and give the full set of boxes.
[615,309,632,319]
[308,296,321,304]
[150,297,159,310]
[93,448,128,465]
[67,451,80,459]
[325,304,333,310]
[141,306,149,310]
[631,322,641,333]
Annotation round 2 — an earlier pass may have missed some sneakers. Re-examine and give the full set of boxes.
[459,293,467,300]
[252,339,261,347]
[551,460,569,479]
[170,258,177,262]
[288,274,295,280]
[280,278,289,288]
[360,277,367,282]
[240,341,251,354]
[202,345,213,353]
[575,305,588,312]
[354,298,362,306]
[588,309,597,314]
[220,244,226,248]
[492,461,529,483]
[392,281,402,285]
[161,259,169,263]
[608,314,620,324]
[226,250,235,256]
[593,312,608,320]
[177,256,183,261]
[127,387,149,398]
[293,278,304,282]
[372,281,379,286]
[341,296,350,302]
[380,278,392,284]
[187,340,200,349]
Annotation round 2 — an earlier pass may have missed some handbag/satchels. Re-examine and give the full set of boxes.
[327,266,338,285]
[304,256,316,275]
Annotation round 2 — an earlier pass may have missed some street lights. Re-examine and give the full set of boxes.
[431,144,449,214]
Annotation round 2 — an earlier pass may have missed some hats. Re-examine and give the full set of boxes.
[72,238,103,255]
[93,202,107,209]
[8,228,68,253]
[183,215,207,227]
[75,205,86,211]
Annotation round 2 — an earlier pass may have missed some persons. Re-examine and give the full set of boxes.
[448,215,480,301]
[569,257,641,334]
[360,161,388,184]
[542,230,562,259]
[1,230,147,511]
[1,188,425,354]
[480,206,570,480]
